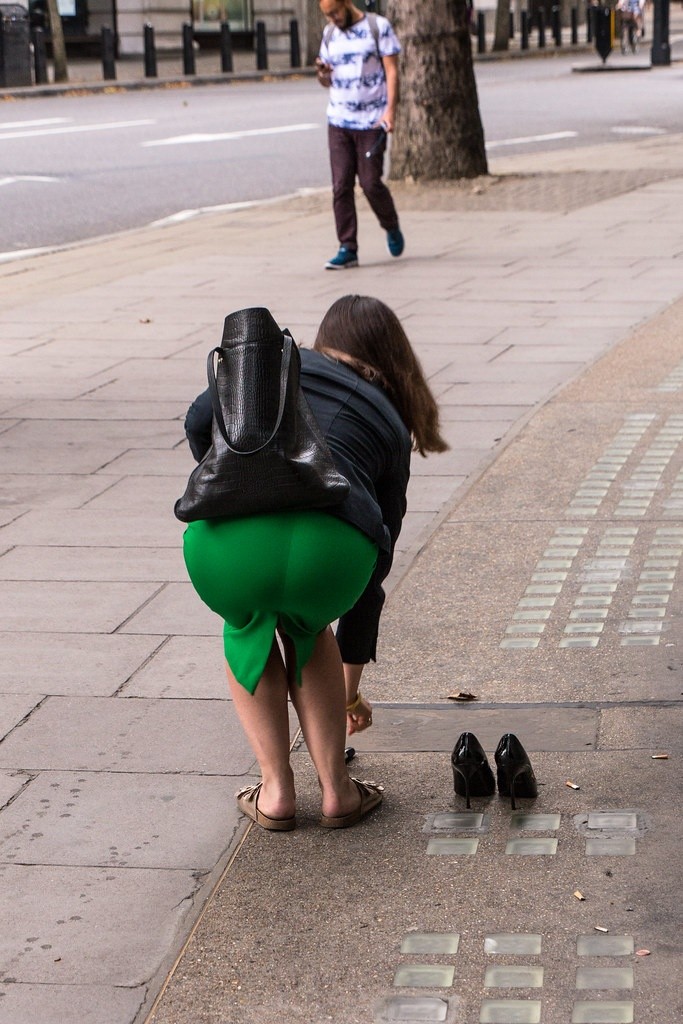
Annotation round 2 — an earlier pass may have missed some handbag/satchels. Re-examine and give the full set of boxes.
[173,307,351,523]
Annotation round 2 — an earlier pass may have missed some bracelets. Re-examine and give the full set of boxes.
[319,71,329,78]
[346,689,362,711]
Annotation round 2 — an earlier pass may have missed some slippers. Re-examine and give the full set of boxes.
[235,783,296,830]
[320,777,383,829]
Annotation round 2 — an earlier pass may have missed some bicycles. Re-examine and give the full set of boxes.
[618,4,644,56]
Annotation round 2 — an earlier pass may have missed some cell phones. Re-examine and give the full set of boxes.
[317,60,334,72]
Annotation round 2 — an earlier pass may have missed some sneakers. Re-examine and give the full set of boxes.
[387,227,404,257]
[325,245,359,269]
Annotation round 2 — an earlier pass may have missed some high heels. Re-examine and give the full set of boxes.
[494,734,538,811]
[451,733,496,809]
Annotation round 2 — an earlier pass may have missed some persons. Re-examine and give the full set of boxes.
[173,295,452,833]
[614,0,645,48]
[315,0,406,271]
